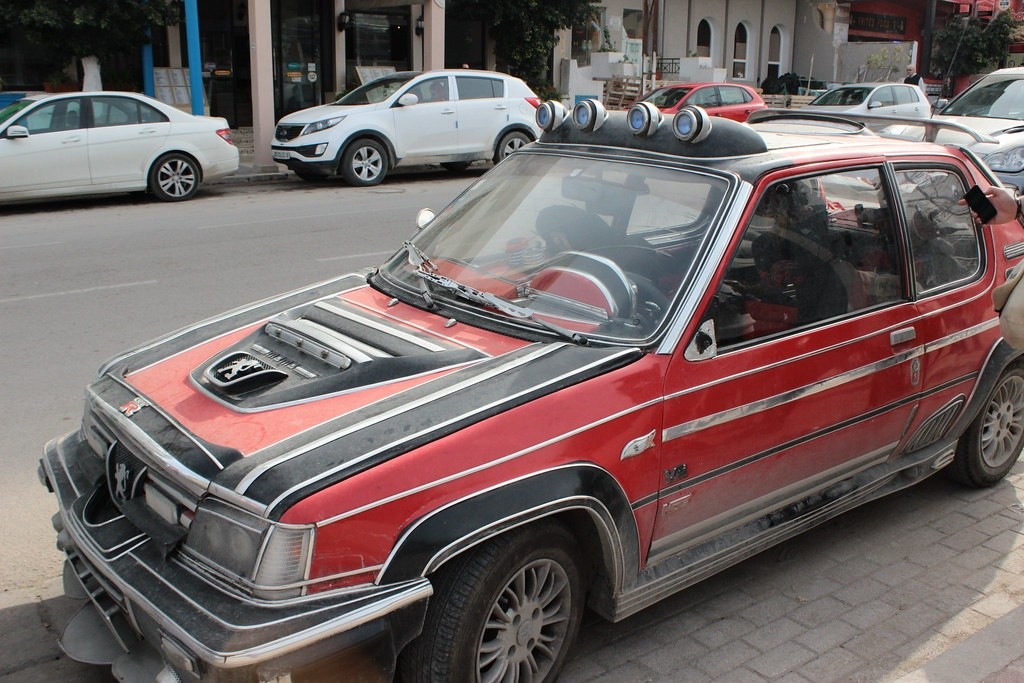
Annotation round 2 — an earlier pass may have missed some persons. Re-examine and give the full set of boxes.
[430,82,447,102]
[959,187,1024,227]
[751,182,844,326]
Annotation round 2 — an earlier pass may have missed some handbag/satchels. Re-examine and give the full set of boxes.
[991,258,1024,350]
[829,259,884,313]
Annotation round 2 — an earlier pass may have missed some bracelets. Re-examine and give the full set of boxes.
[1015,198,1022,220]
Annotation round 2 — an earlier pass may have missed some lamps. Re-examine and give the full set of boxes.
[416,14,424,37]
[338,9,350,31]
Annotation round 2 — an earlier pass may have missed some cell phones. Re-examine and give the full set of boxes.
[963,184,997,224]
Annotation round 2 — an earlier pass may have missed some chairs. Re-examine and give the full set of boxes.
[849,91,863,105]
[535,205,610,263]
[65,111,77,130]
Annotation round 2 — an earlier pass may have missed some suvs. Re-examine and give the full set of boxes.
[271,69,548,187]
[800,82,932,121]
[621,82,769,124]
[36,98,1023,683]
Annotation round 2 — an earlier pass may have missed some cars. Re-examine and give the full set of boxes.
[0,91,239,203]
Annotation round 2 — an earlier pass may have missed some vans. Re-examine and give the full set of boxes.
[929,67,1024,196]
